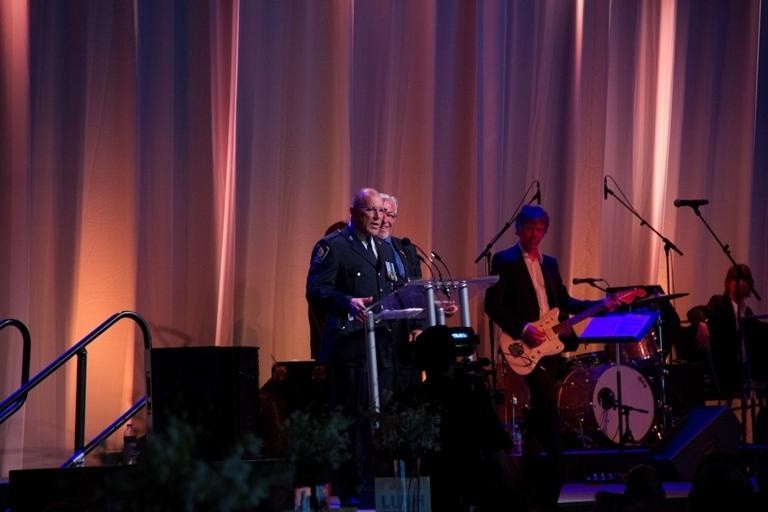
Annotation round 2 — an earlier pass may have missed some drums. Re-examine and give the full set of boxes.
[559,360,655,446]
[605,325,660,363]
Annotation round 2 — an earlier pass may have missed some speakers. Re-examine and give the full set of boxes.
[144,346,259,460]
[654,404,742,483]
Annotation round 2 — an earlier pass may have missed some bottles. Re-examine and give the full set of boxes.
[513,424,524,456]
[122,424,138,467]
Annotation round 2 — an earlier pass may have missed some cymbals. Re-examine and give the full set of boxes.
[632,293,690,305]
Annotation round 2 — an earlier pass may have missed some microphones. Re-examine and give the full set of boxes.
[416,252,437,282]
[430,251,453,281]
[674,199,709,207]
[401,237,444,281]
[597,387,615,410]
[604,176,607,199]
[537,183,541,205]
[572,278,603,285]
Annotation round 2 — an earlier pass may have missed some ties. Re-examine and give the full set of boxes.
[364,235,377,259]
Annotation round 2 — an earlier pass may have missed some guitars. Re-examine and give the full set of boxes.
[500,289,647,375]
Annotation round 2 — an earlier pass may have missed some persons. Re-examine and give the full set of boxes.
[684,263,759,390]
[305,186,458,423]
[431,454,768,511]
[483,203,618,450]
[313,190,432,412]
[310,220,350,360]
